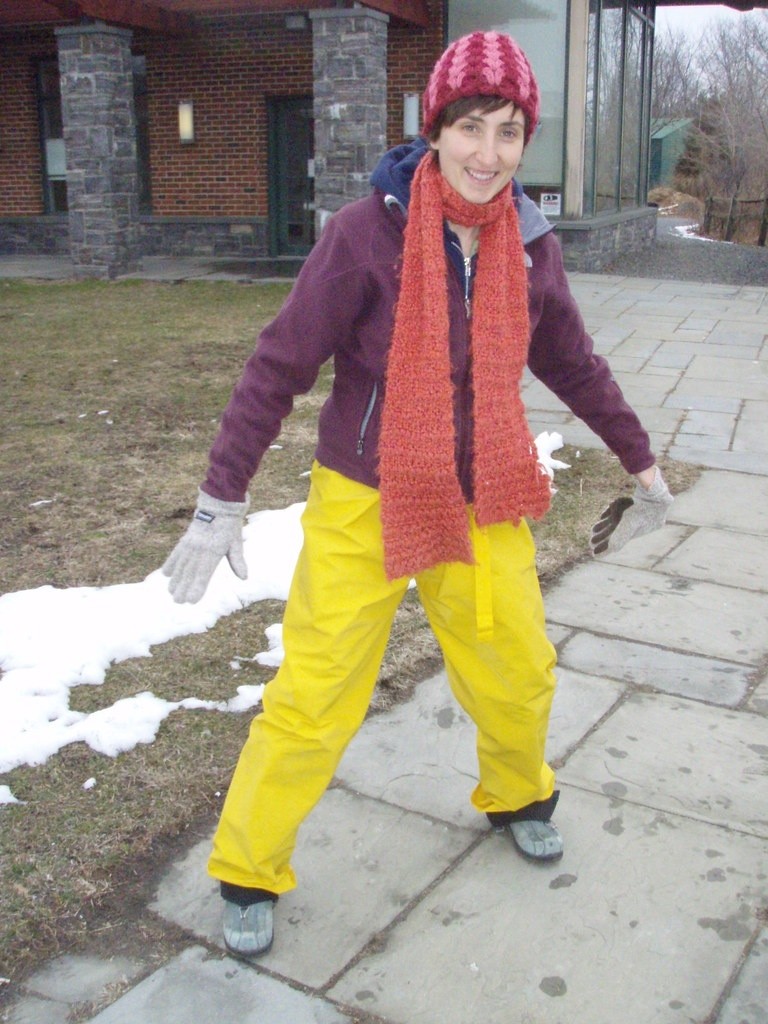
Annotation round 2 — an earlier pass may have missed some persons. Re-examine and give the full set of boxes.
[161,29,671,962]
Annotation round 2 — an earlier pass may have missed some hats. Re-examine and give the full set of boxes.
[421,31,539,146]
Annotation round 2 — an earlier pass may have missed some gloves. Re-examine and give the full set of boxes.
[590,466,674,560]
[162,485,251,604]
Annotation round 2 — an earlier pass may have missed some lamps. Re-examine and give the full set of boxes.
[177,101,197,146]
[403,94,420,141]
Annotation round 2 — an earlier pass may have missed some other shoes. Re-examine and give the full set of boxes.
[503,819,564,859]
[221,898,274,959]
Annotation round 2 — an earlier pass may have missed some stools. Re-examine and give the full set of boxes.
[213,229,255,258]
